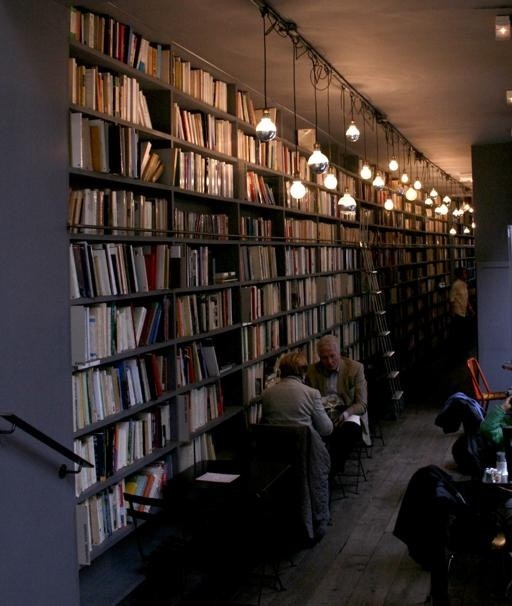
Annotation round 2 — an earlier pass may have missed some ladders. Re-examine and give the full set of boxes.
[359,241,408,424]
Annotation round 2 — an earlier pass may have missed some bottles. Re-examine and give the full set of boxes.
[482,467,502,484]
[496,451,510,484]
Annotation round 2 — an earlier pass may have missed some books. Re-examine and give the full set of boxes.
[68,7,474,568]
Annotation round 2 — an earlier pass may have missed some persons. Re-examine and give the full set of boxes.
[480,394,512,446]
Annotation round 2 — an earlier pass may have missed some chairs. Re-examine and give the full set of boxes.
[467,357,507,415]
